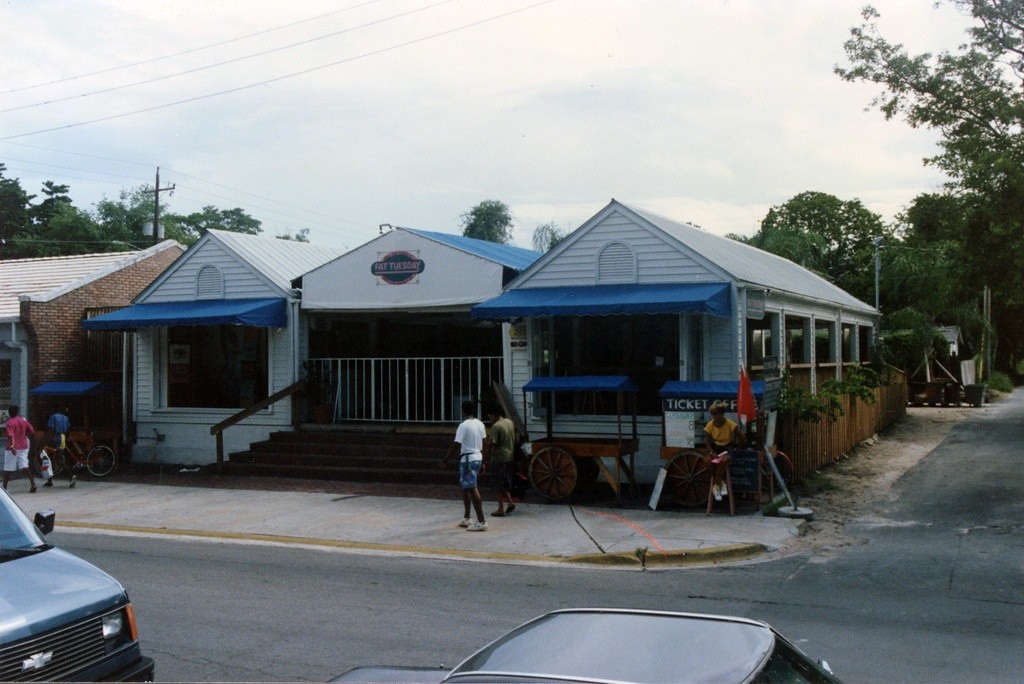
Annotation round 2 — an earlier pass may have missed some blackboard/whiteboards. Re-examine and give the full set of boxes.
[728,449,762,493]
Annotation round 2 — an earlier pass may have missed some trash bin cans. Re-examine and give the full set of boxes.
[964,384,984,407]
[924,382,948,407]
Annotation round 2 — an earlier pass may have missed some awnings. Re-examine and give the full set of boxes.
[471,282,731,323]
[83,297,287,332]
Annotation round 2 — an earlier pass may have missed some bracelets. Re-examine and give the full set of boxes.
[710,452,712,454]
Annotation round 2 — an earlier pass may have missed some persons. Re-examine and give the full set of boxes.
[487,404,516,516]
[43,405,77,488]
[2,405,37,493]
[704,403,744,501]
[439,401,488,530]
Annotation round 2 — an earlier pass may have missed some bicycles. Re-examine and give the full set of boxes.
[34,429,117,478]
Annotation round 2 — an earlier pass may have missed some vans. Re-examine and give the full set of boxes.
[1,485,156,682]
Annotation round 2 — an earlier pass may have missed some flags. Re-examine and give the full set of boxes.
[738,367,755,421]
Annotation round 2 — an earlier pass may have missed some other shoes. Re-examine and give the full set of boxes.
[505,504,515,513]
[43,482,53,486]
[467,520,488,531]
[712,486,722,500]
[29,484,37,493]
[69,474,77,488]
[456,518,473,527]
[491,511,504,516]
[720,483,728,495]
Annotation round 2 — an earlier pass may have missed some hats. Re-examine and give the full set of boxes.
[709,400,727,413]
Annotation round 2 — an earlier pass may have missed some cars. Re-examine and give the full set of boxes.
[324,607,845,684]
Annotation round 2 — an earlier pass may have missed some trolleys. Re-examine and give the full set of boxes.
[512,376,638,505]
[660,382,793,506]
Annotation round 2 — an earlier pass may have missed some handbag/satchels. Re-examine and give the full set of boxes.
[46,416,61,448]
[58,433,66,449]
[41,455,53,480]
